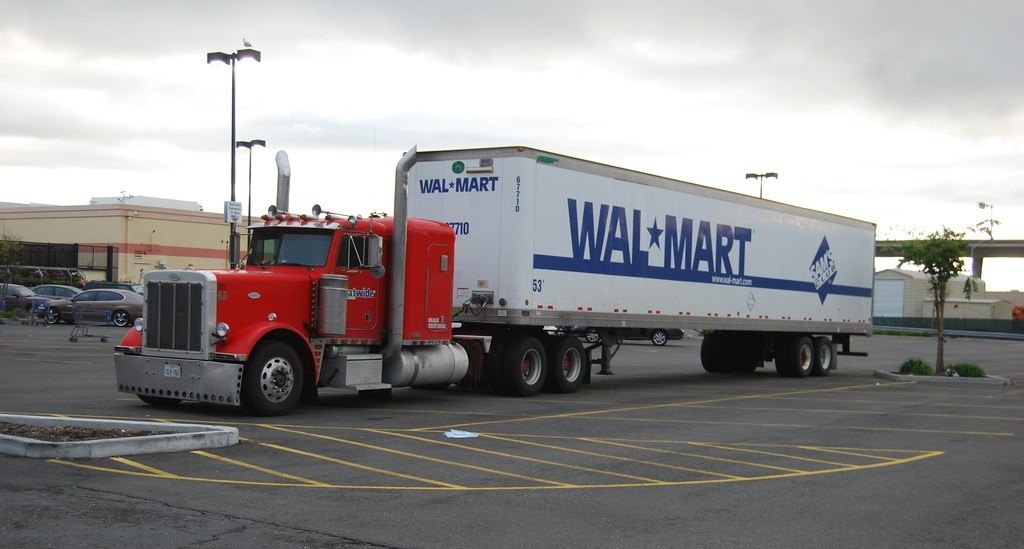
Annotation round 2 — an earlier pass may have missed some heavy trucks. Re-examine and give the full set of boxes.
[113,147,877,418]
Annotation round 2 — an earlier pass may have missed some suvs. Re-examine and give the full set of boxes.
[0,283,47,316]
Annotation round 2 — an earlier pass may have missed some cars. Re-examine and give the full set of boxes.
[36,288,144,327]
[567,326,685,346]
[31,285,86,304]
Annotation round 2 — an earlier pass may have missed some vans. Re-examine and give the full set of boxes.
[80,281,143,297]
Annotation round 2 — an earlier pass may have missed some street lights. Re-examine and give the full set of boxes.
[207,49,261,269]
[746,172,778,199]
[236,139,266,255]
